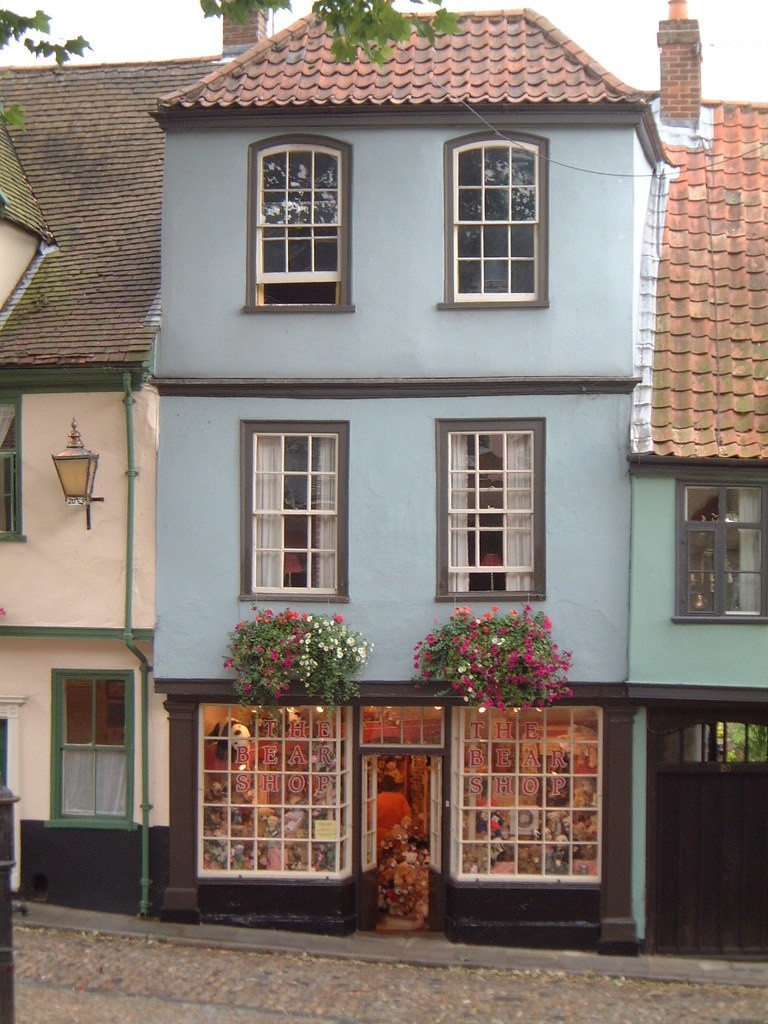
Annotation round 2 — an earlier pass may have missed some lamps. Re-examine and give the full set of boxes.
[50,417,106,529]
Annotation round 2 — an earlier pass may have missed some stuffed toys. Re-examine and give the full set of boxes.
[376,815,430,919]
[462,723,598,875]
[205,708,335,873]
[368,760,385,780]
[385,761,404,783]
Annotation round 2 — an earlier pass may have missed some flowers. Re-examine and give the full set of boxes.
[213,601,373,714]
[402,606,577,713]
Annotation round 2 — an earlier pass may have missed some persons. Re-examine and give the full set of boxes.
[368,775,413,864]
